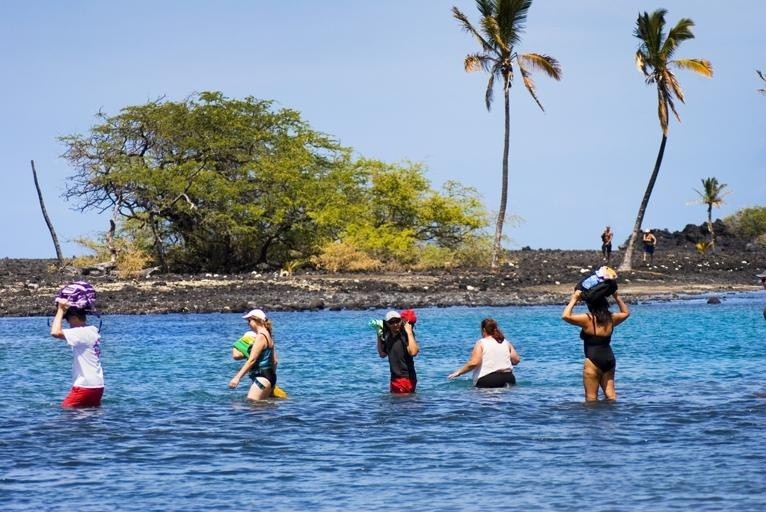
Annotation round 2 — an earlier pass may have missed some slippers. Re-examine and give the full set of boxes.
[55,280,97,313]
[575,267,618,300]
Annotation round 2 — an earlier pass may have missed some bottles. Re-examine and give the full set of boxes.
[242,308,267,323]
[384,311,402,320]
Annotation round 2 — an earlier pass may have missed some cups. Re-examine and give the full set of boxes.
[389,319,400,324]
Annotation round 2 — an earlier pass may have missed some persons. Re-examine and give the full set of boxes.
[602,226,613,262]
[50,295,104,407]
[643,228,656,262]
[228,309,277,400]
[377,311,419,394]
[561,288,630,401]
[448,318,520,388]
